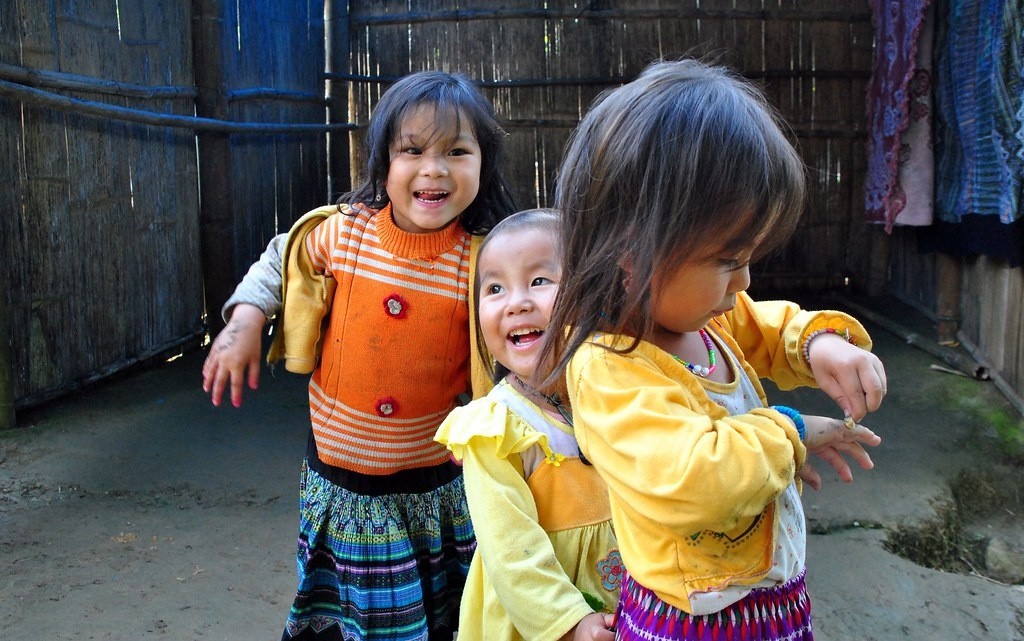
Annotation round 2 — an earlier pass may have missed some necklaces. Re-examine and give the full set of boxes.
[671,328,716,377]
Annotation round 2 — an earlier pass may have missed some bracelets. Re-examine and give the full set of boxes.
[770,404,805,444]
[803,328,857,370]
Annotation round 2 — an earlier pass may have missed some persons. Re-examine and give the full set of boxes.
[202,71,519,641]
[434,208,625,641]
[528,61,886,641]
